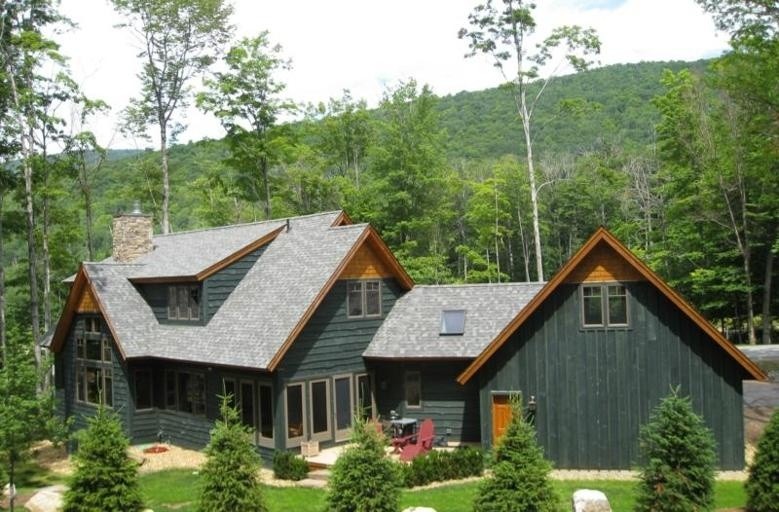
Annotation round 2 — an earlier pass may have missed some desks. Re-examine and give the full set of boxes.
[390,433,418,455]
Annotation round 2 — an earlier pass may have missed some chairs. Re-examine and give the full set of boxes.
[394,419,435,462]
